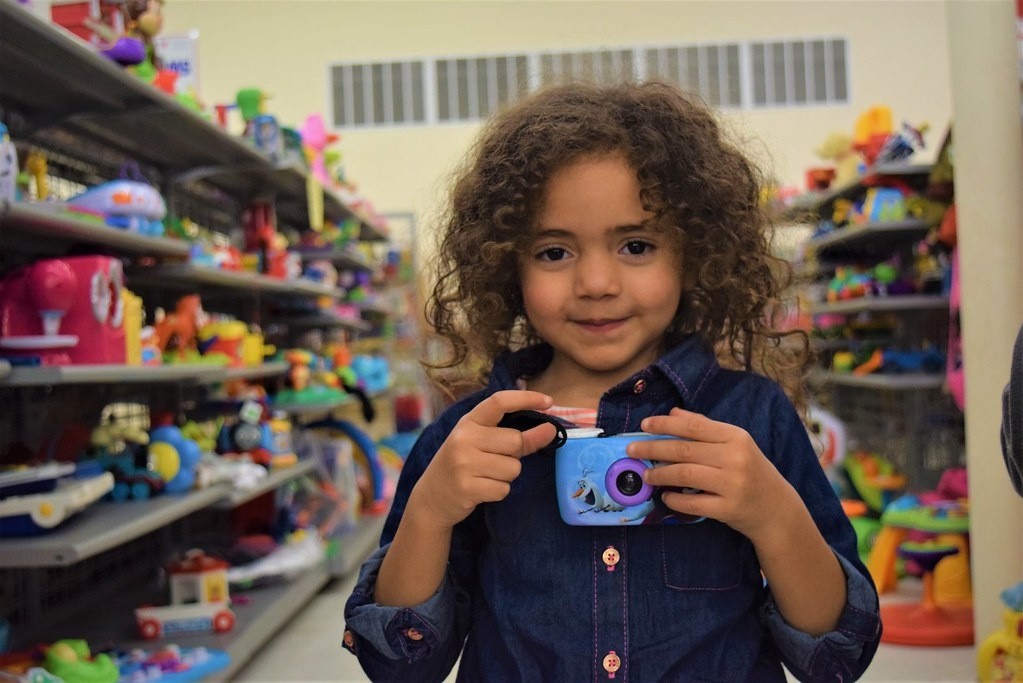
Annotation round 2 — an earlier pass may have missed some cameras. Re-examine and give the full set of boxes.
[556,431,709,527]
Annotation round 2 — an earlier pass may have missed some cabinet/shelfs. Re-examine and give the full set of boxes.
[0,1,404,683]
[798,163,952,393]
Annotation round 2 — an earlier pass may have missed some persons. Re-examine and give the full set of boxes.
[341,80,883,683]
[1000,323,1022,497]
